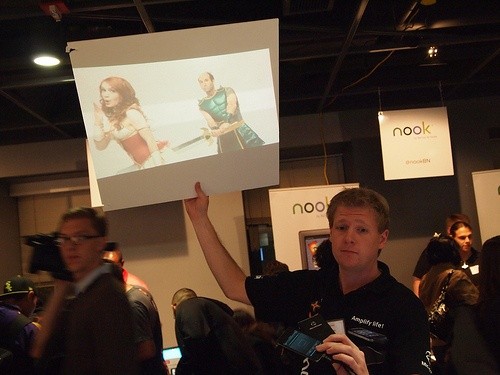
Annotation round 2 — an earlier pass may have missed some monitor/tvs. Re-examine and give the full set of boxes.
[162,347,182,375]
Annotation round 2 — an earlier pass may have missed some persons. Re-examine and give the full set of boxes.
[171,238,338,375]
[197,72,265,155]
[0,208,168,375]
[93,77,179,169]
[184,182,429,375]
[412,214,500,375]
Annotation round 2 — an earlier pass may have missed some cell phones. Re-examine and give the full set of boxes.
[276,327,328,362]
[297,315,337,343]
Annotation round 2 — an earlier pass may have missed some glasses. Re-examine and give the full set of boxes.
[55,234,100,246]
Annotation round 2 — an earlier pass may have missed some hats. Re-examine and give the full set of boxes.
[0,274,34,298]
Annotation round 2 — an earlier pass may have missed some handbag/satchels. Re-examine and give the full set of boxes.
[427,270,457,343]
